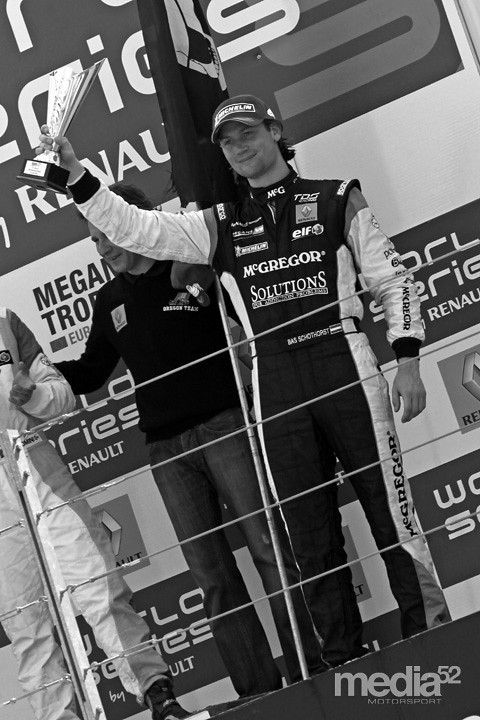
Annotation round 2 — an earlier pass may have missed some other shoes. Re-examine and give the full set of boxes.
[149,685,191,720]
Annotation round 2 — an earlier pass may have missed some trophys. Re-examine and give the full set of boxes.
[15,57,107,195]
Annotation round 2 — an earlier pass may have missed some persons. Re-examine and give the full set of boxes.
[0,303,194,720]
[35,93,454,670]
[50,181,329,703]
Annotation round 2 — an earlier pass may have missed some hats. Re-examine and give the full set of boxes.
[209,94,279,146]
